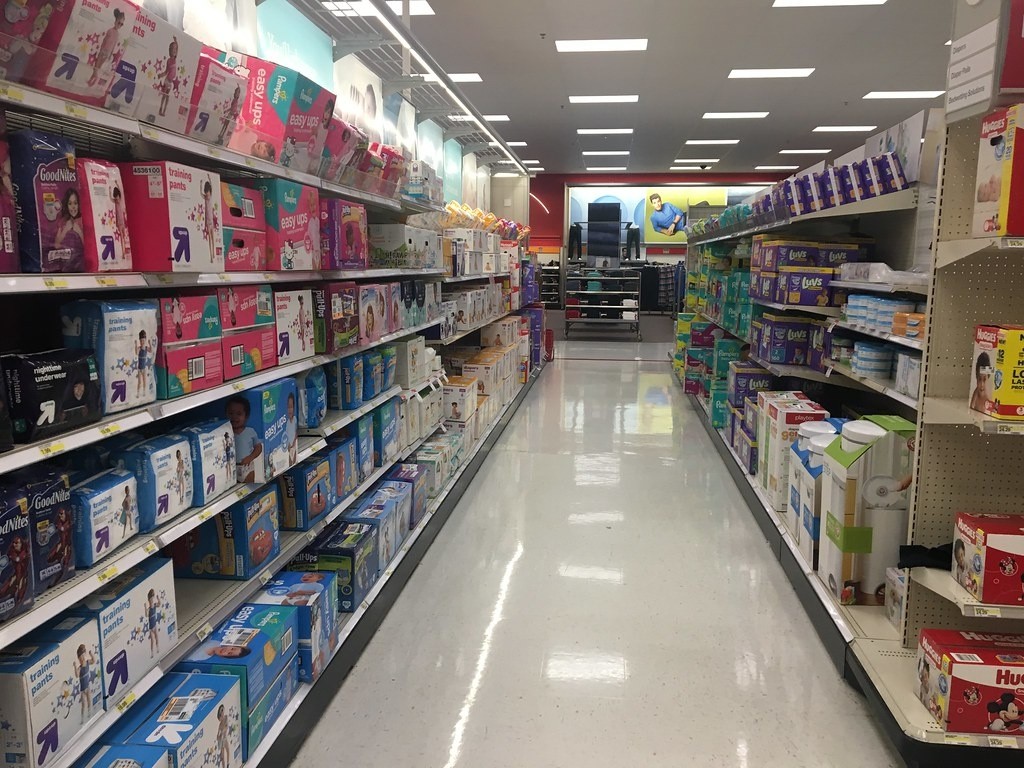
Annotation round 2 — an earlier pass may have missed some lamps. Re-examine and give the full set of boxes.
[290,1,530,178]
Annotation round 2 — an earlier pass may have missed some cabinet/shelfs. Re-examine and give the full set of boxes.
[541,261,642,341]
[146,117,372,768]
[0,75,260,768]
[423,272,521,455]
[297,177,466,609]
[846,0,1023,767]
[781,98,944,768]
[666,106,869,680]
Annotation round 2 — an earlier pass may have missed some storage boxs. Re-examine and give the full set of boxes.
[1,0,548,767]
[673,103,1023,737]
